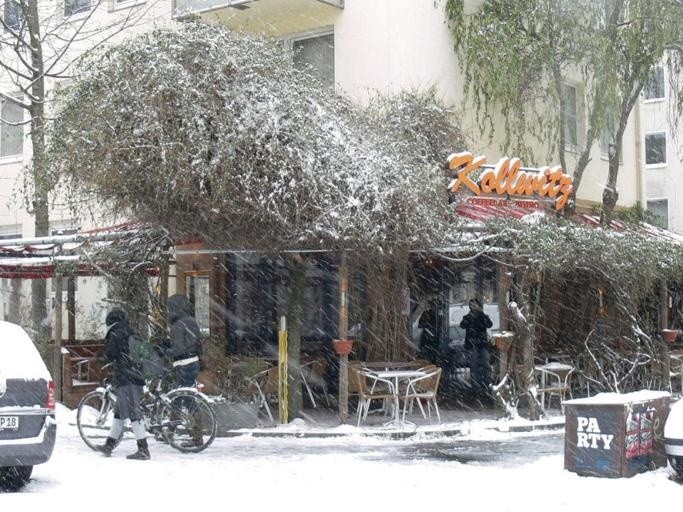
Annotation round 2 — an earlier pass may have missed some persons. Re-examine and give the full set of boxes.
[151,293,204,446]
[460,299,494,403]
[416,299,443,359]
[96,306,151,461]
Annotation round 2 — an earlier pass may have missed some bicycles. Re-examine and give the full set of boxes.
[75,357,219,453]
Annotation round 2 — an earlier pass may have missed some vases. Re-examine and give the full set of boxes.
[662,331,677,343]
[492,331,515,352]
[332,340,352,355]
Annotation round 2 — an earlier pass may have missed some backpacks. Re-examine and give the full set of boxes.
[113,328,163,382]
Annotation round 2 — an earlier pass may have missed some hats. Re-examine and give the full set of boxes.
[105,305,130,327]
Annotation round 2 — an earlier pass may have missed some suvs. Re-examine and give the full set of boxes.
[0,319,56,491]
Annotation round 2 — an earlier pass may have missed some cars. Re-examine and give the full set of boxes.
[663,396,683,480]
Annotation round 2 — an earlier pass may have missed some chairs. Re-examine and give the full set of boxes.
[218,358,442,428]
[534,361,575,415]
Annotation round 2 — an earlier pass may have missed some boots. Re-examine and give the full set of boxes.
[126,438,151,460]
[180,410,204,448]
[157,408,182,443]
[95,435,116,457]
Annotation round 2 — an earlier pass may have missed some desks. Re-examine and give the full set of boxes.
[563,395,671,479]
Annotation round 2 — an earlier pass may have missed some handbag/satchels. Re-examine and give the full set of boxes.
[177,319,210,372]
